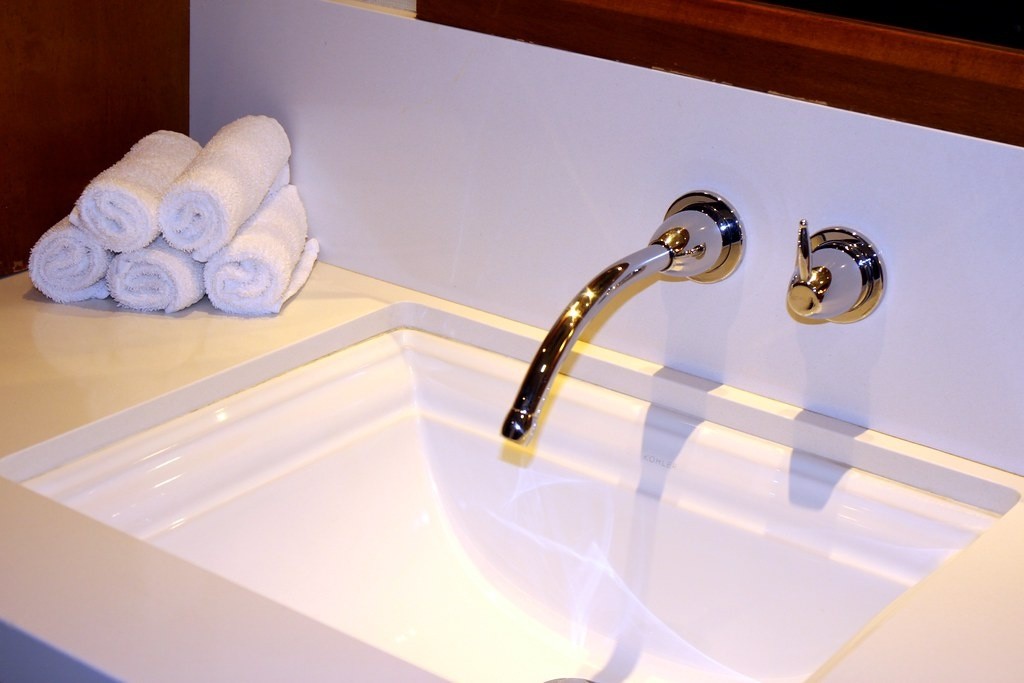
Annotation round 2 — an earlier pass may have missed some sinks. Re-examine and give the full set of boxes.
[1,300,1022,683]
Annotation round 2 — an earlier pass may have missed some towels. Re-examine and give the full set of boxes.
[26,114,320,318]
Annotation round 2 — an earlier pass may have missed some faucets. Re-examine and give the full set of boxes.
[500,207,731,449]
